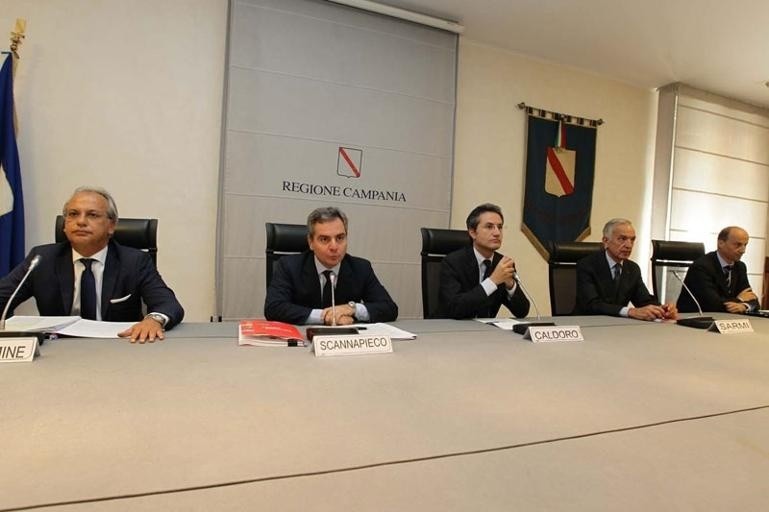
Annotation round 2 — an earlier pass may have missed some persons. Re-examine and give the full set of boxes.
[676,227,761,315]
[570,218,679,321]
[0,185,185,344]
[429,204,530,320]
[263,206,398,327]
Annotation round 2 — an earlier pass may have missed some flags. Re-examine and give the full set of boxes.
[0,49,24,285]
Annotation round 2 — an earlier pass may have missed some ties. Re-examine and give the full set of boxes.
[483,260,492,282]
[322,271,332,309]
[723,266,733,294]
[78,258,97,321]
[613,263,622,294]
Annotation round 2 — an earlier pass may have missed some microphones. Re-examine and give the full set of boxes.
[513,271,555,335]
[0,254,45,346]
[672,270,717,329]
[306,272,359,342]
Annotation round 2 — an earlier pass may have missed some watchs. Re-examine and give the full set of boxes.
[143,313,166,326]
[348,301,356,317]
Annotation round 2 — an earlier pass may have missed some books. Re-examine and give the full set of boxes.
[238,321,306,347]
[0,315,143,339]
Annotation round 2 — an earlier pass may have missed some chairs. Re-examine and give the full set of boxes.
[549,241,604,316]
[55,215,158,278]
[421,228,473,319]
[651,239,705,307]
[266,222,311,296]
